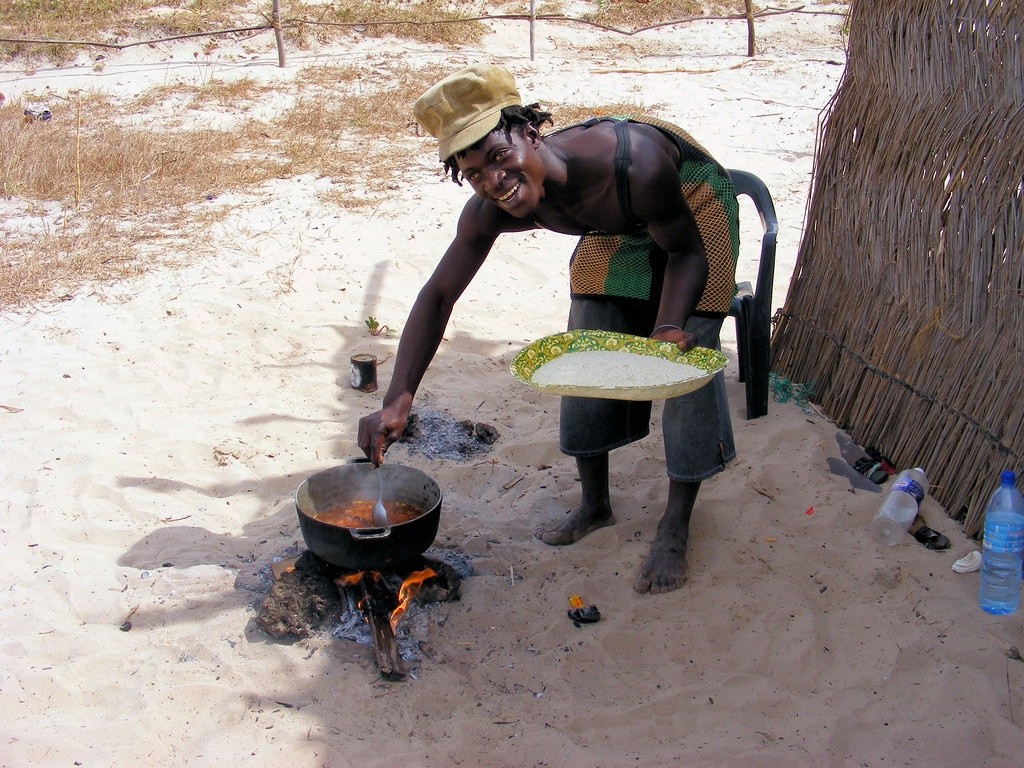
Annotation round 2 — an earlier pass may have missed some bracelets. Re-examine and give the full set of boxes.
[650,325,680,338]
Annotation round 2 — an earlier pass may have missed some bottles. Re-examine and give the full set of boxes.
[869,467,929,546]
[977,471,1024,616]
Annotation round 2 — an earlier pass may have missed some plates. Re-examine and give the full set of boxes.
[509,328,730,401]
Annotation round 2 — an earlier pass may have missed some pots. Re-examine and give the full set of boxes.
[294,456,444,570]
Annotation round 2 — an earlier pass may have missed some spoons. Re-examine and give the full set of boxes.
[371,463,388,527]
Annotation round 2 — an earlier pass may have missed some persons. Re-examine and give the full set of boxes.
[358,61,738,593]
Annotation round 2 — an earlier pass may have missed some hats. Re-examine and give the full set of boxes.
[412,65,522,162]
